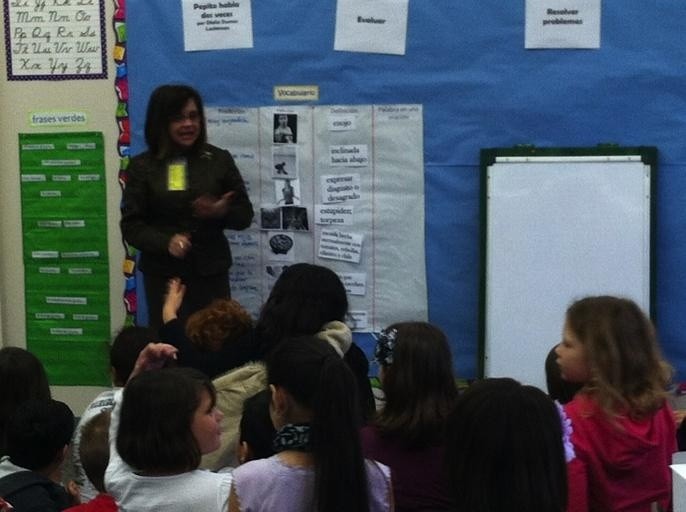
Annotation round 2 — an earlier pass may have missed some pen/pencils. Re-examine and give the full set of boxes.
[179,240,183,249]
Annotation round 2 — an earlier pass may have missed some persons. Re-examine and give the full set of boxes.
[119,82,256,327]
[1,264,395,510]
[274,159,290,174]
[365,295,686,512]
[274,112,293,142]
[276,178,300,204]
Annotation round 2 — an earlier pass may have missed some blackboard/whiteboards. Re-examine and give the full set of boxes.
[477,145,659,396]
